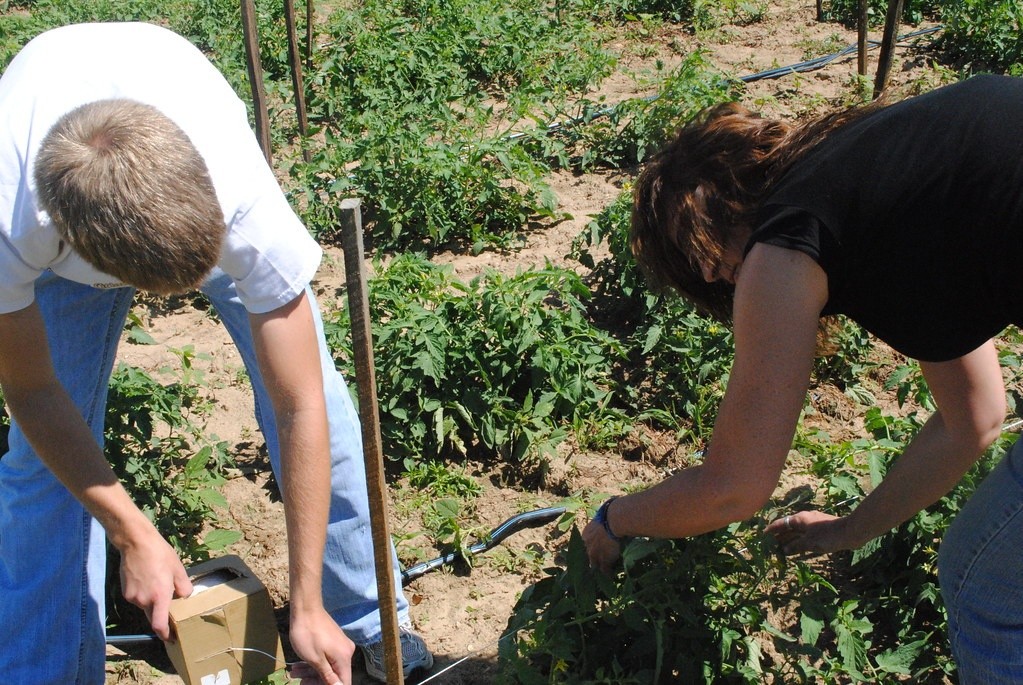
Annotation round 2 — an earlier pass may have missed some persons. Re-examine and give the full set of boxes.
[0,21,434,685]
[583,77,1023,684]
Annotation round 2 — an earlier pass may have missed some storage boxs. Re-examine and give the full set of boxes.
[162,554,287,685]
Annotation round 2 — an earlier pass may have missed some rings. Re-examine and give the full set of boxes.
[784,516,793,531]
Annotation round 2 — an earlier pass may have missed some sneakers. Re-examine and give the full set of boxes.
[359,621,433,682]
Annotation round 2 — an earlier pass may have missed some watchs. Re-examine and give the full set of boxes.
[596,496,626,542]
